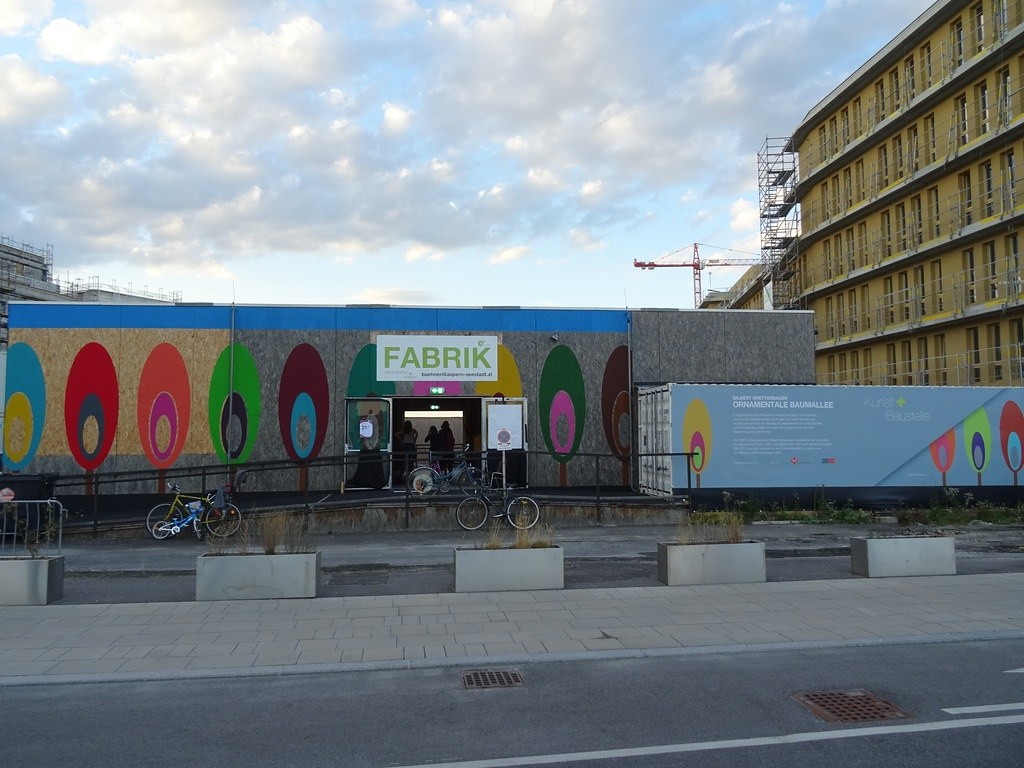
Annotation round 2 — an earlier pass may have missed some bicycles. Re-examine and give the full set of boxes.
[152,501,204,541]
[146,482,242,537]
[408,443,489,497]
[456,471,542,530]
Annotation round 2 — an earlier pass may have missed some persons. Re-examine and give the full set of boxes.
[347,392,386,490]
[425,421,455,485]
[395,420,418,475]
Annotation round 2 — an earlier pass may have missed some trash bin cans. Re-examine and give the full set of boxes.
[487,451,526,490]
[0,472,59,543]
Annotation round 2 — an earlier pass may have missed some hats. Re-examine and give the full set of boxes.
[0,488,14,502]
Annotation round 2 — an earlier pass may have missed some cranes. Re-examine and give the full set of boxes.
[634,242,761,309]
